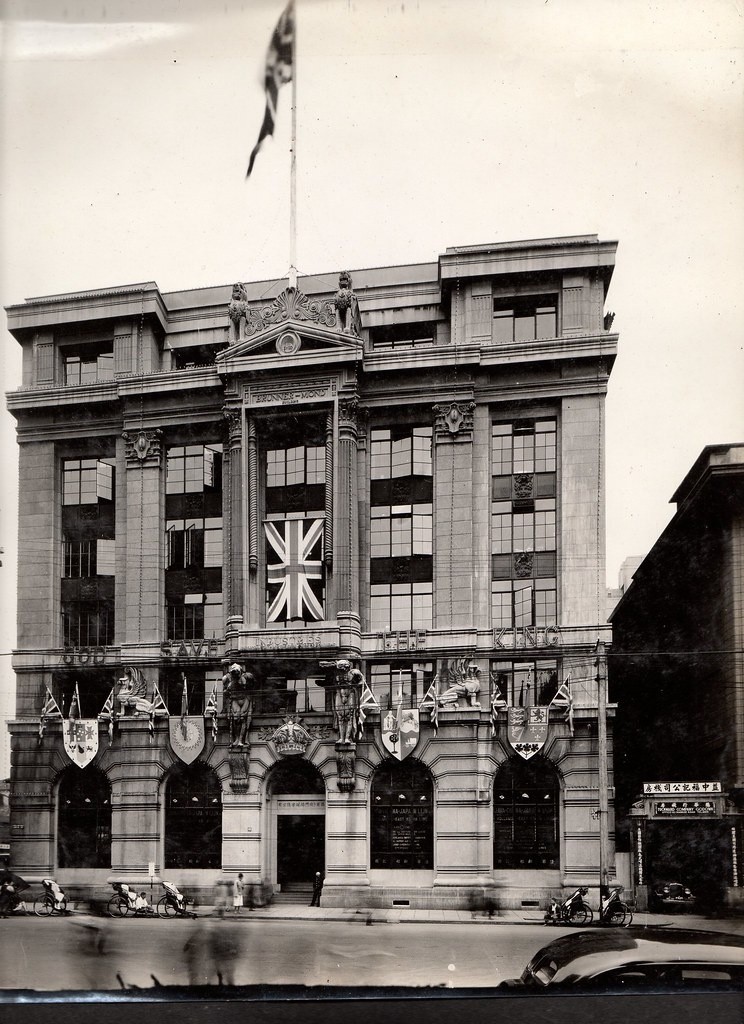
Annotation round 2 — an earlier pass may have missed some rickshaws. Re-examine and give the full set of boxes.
[588,884,635,928]
[33,878,96,918]
[0,868,39,919]
[105,881,159,918]
[156,881,218,918]
[522,886,593,927]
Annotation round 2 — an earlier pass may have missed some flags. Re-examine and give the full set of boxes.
[150,687,168,740]
[99,693,114,745]
[358,683,377,734]
[246,0,294,176]
[39,691,61,737]
[265,519,325,622]
[68,692,79,741]
[424,682,439,732]
[490,686,505,734]
[206,686,218,740]
[553,678,574,716]
[180,681,189,740]
[524,683,531,727]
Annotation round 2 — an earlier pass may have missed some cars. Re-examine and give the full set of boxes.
[652,882,697,910]
[497,925,743,991]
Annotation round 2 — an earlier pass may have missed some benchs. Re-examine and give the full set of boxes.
[599,890,619,916]
[121,884,137,900]
[560,888,582,912]
[165,882,183,902]
[48,882,65,901]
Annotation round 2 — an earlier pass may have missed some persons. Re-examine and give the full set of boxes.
[334,662,354,743]
[222,665,252,746]
[135,892,154,915]
[308,872,325,907]
[0,879,29,915]
[234,874,245,914]
[543,897,561,926]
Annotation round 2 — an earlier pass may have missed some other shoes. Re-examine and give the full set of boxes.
[249,909,255,911]
[234,911,237,913]
[308,904,315,907]
[317,905,320,907]
[239,912,243,914]
